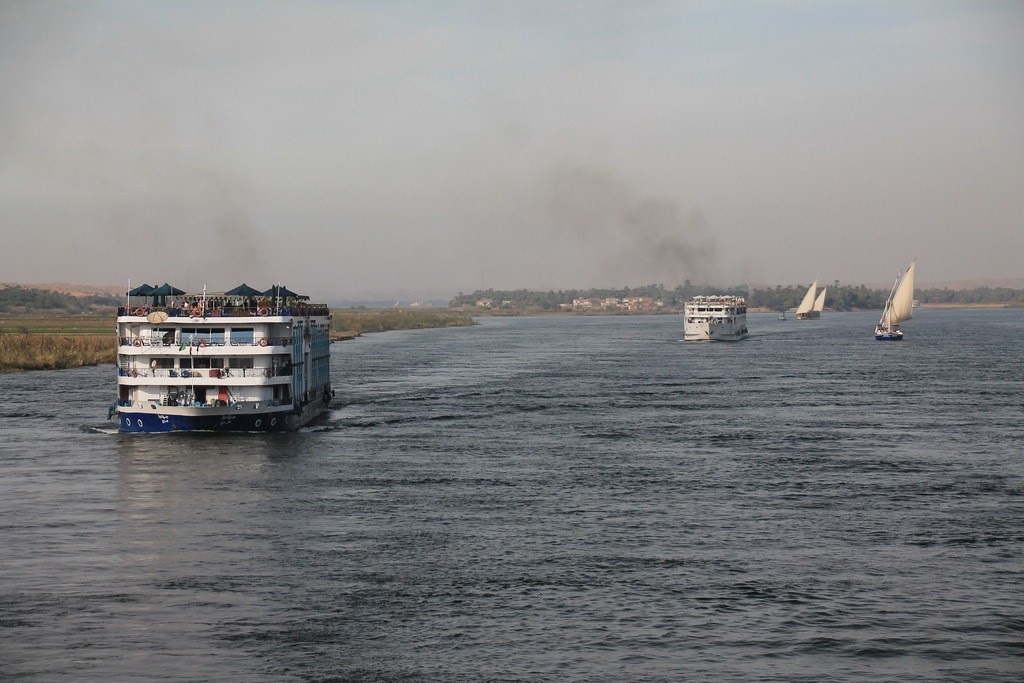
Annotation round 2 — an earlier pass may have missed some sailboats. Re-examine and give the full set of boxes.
[874,256,917,340]
[796,280,826,319]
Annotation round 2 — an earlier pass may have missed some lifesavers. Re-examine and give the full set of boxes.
[198,339,206,347]
[193,307,202,317]
[259,338,268,347]
[259,307,269,317]
[135,307,145,317]
[133,339,141,347]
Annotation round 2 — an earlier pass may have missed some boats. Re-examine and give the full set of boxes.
[684,295,748,341]
[778,316,787,321]
[107,278,335,432]
